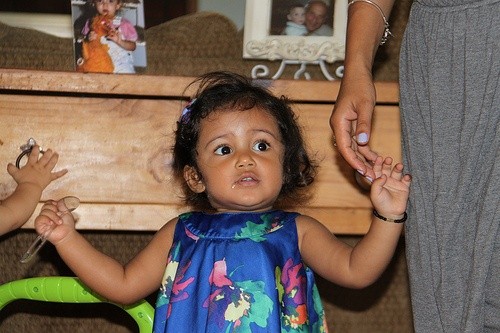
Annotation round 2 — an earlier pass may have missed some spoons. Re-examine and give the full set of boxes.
[19,196,80,264]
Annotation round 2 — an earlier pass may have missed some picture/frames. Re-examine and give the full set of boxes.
[242,0,348,63]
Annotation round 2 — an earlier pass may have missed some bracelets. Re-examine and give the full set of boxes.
[372,209,408,223]
[349,0,390,46]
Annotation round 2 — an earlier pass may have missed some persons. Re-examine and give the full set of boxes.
[282,0,332,35]
[0,143,69,237]
[34,74,410,333]
[77,0,138,73]
[329,0,500,333]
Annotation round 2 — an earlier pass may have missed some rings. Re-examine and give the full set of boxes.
[333,136,336,146]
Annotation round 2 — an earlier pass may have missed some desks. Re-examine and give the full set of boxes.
[0,70,406,234]
